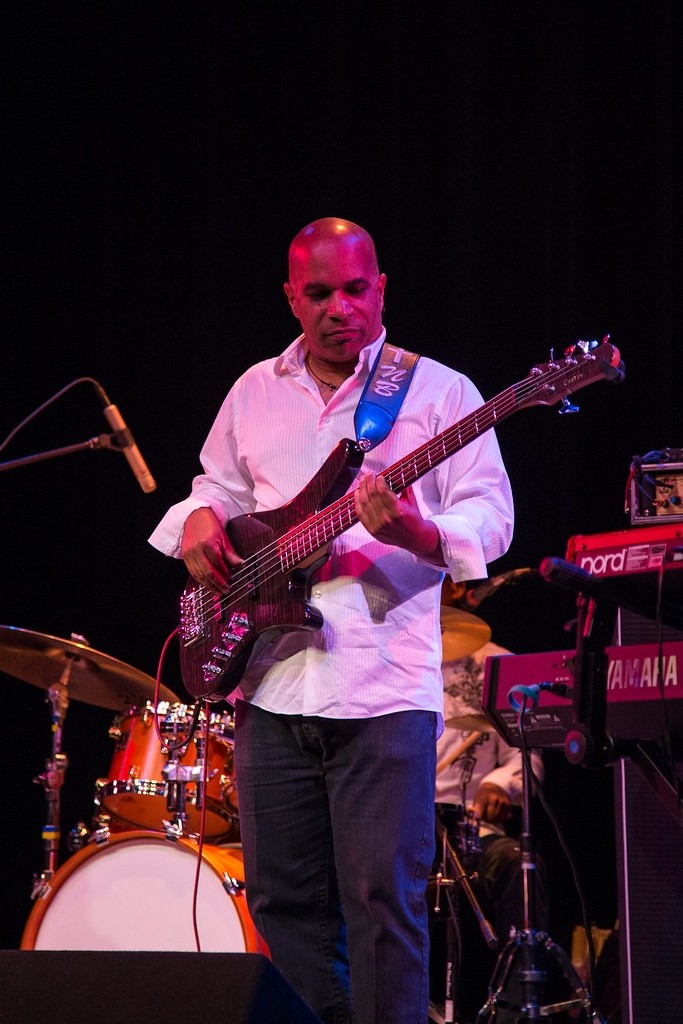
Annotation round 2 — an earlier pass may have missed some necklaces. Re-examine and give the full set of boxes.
[307,350,342,390]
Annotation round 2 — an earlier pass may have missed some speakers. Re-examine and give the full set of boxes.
[611,579,683,1024]
[0,950,323,1024]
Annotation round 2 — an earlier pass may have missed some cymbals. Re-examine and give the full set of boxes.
[440,605,491,664]
[0,629,175,712]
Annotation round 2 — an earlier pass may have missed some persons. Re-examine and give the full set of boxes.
[148,218,514,1024]
[428,559,547,1005]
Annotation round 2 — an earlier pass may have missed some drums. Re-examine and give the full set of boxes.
[95,696,239,842]
[431,802,481,886]
[18,830,271,951]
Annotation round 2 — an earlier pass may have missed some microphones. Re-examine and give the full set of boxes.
[166,734,185,812]
[195,725,206,812]
[95,383,156,493]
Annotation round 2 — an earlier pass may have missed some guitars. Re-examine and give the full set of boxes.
[179,332,623,700]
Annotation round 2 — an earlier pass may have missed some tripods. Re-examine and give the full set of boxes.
[474,747,611,1024]
